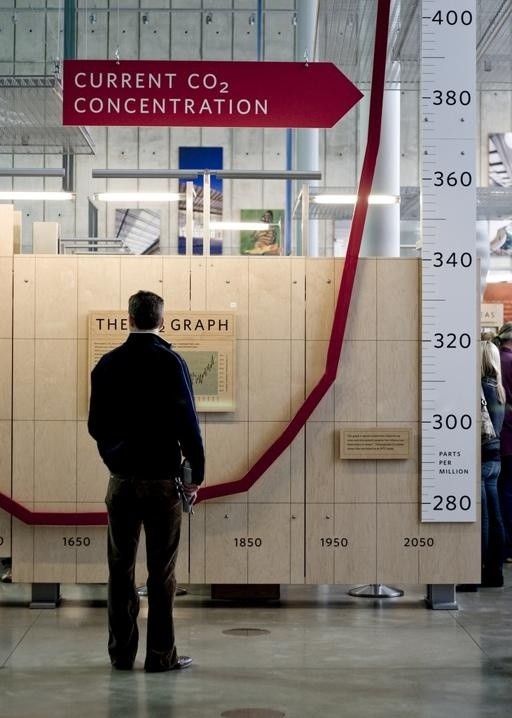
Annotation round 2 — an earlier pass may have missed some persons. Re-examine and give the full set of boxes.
[87,289,207,674]
[247,210,279,256]
[455,321,512,593]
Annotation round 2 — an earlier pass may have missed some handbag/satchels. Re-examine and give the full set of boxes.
[480,399,497,446]
[181,459,193,512]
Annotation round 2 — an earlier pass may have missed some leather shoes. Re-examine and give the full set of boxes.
[175,655,192,670]
